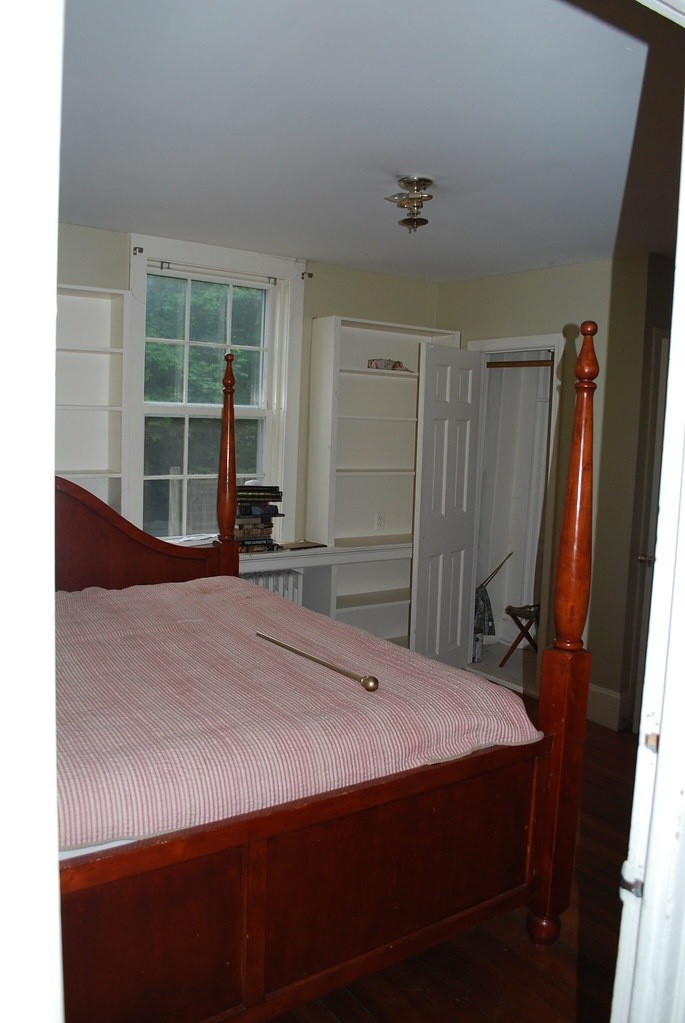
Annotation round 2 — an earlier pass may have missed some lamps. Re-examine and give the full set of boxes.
[384,178,433,234]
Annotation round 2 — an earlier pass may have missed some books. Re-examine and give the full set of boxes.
[234,485,285,552]
[279,539,327,551]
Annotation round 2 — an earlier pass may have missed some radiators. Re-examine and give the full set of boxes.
[240,570,302,605]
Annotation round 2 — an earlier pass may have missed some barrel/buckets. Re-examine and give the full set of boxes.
[472,628,483,662]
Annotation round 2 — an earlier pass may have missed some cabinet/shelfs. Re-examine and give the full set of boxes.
[303,561,411,653]
[305,316,459,547]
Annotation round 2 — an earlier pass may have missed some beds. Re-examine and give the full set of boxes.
[56,328,604,1023]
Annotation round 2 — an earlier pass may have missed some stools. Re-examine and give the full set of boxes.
[500,603,540,668]
